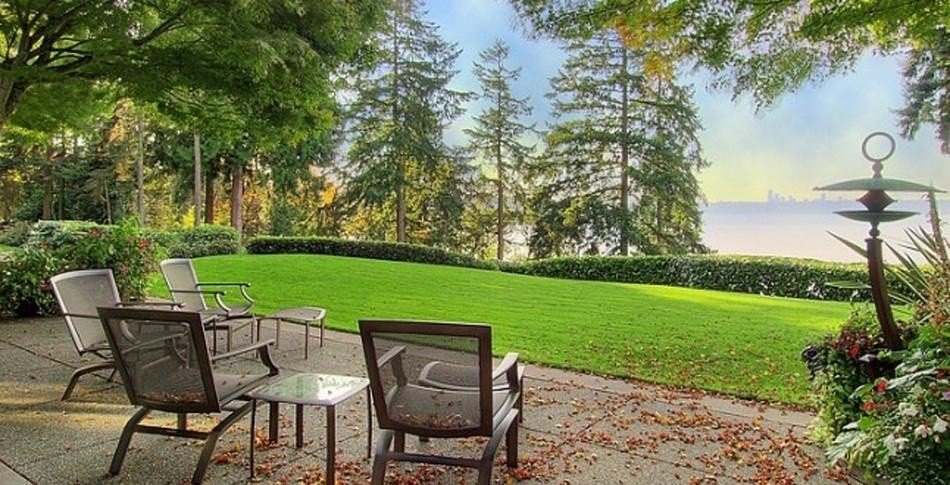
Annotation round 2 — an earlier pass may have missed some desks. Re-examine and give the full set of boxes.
[263,305,326,360]
[249,371,374,485]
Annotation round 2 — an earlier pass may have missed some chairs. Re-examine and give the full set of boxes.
[50,267,190,403]
[96,305,281,484]
[158,258,263,352]
[358,318,527,483]
